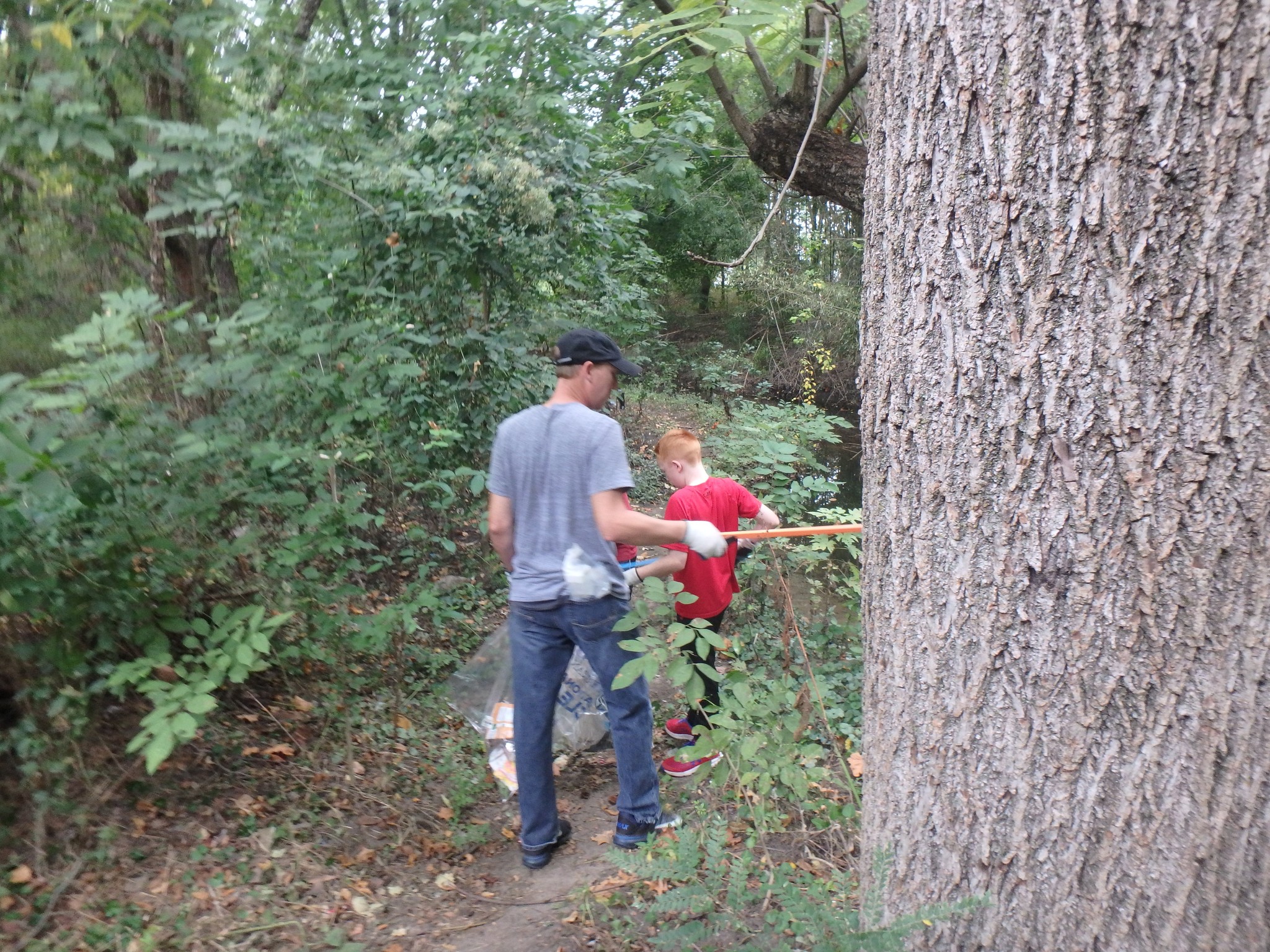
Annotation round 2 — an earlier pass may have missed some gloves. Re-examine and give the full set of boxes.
[623,567,643,586]
[680,521,729,560]
[735,538,756,563]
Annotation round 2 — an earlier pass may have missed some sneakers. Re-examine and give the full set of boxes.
[662,716,725,778]
[613,810,685,850]
[522,819,572,870]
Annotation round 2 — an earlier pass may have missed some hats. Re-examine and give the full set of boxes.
[553,327,643,377]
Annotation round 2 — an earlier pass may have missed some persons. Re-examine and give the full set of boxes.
[621,426,779,779]
[482,327,728,871]
